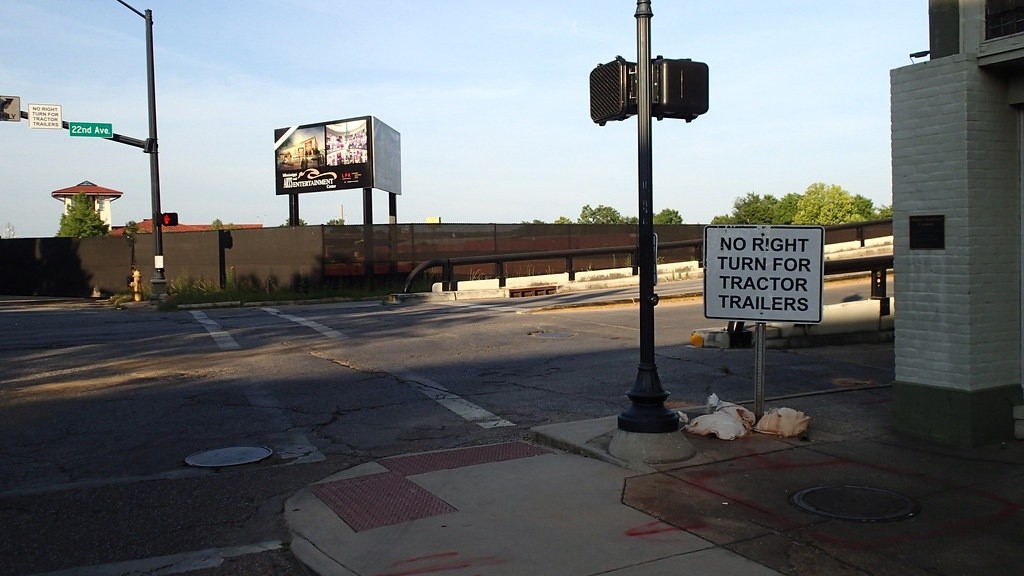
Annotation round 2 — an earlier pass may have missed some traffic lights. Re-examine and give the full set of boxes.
[225,231,233,250]
[161,213,178,226]
[589,58,637,126]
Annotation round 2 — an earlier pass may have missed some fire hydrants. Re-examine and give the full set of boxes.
[128,269,142,303]
